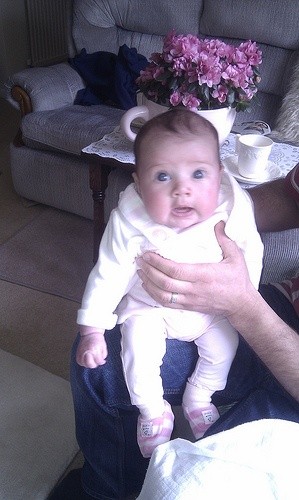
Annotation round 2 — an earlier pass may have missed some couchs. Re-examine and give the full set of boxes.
[170,225,299,446]
[0,0,299,226]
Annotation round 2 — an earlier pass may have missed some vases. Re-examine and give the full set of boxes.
[118,94,237,151]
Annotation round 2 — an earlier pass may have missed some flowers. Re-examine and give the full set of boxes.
[135,28,264,113]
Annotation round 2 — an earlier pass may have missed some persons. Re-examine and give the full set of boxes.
[55,165,299,500]
[77,109,264,458]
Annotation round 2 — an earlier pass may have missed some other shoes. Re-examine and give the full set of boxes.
[136,399,175,457]
[180,402,220,439]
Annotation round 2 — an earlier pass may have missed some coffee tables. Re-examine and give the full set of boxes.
[80,122,299,268]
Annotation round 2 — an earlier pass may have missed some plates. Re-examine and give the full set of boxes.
[221,155,282,185]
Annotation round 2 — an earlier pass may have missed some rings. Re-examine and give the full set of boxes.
[170,293,178,306]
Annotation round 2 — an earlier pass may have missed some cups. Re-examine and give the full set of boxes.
[235,133,274,179]
